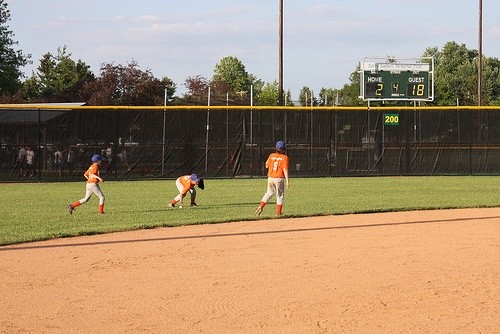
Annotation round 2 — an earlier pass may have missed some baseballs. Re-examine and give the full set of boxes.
[179,206,182,208]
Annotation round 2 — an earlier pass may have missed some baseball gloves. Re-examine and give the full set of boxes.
[198,179,204,190]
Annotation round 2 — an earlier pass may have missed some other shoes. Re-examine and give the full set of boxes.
[275,213,286,217]
[254,206,262,216]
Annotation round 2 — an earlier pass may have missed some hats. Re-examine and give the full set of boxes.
[190,174,200,182]
[92,155,105,162]
[276,141,285,149]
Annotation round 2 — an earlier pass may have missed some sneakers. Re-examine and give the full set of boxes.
[169,203,176,209]
[68,204,74,214]
[98,212,106,215]
[190,202,197,207]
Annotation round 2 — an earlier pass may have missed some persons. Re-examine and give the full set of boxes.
[68,154,108,215]
[254,141,290,218]
[168,173,205,209]
[5,137,129,179]
